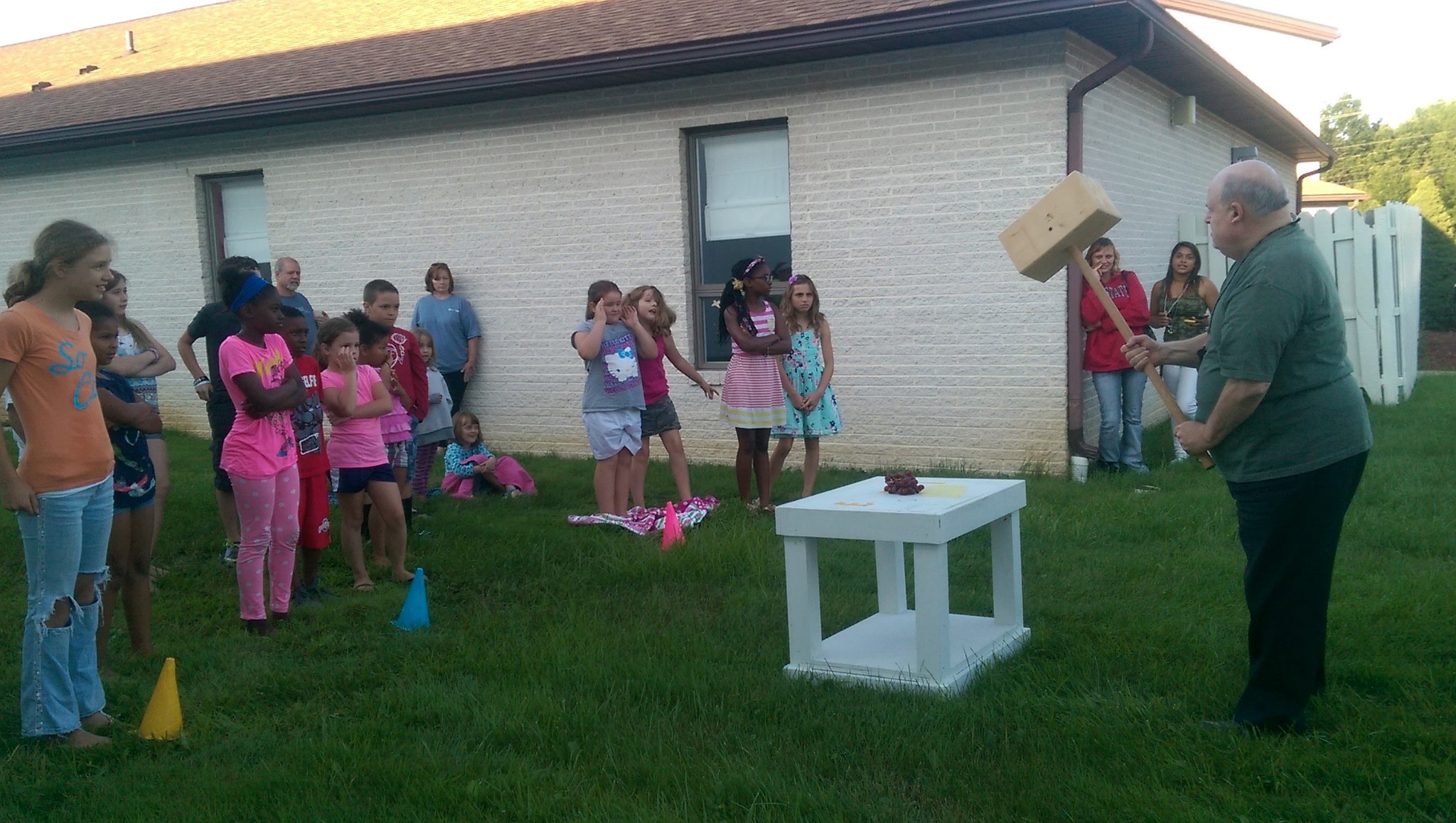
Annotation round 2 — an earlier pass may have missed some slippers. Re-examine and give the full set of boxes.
[352,580,380,593]
[391,578,415,586]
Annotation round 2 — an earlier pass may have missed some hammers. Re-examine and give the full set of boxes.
[998,171,1217,472]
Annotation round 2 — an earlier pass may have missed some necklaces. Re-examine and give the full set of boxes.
[1162,278,1195,333]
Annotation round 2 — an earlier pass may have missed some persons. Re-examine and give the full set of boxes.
[719,253,844,517]
[1120,159,1374,735]
[1149,240,1218,472]
[178,257,538,641]
[1081,234,1151,476]
[1,219,178,751]
[571,280,719,518]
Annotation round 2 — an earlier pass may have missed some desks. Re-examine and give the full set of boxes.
[775,476,1031,701]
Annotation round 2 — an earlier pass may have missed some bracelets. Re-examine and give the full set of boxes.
[193,376,209,387]
[146,347,159,360]
[386,361,390,367]
[764,347,769,357]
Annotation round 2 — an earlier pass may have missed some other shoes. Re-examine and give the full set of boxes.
[247,618,274,636]
[505,488,524,500]
[224,544,242,563]
[1199,717,1251,735]
[271,611,292,624]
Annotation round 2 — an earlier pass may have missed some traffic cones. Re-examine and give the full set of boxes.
[660,500,685,553]
[126,656,184,743]
[389,566,432,632]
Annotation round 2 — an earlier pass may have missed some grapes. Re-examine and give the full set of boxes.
[884,470,925,495]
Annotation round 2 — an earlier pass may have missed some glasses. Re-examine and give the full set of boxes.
[749,275,773,282]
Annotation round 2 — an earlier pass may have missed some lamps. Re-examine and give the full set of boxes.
[1172,96,1195,125]
[1231,147,1258,164]
